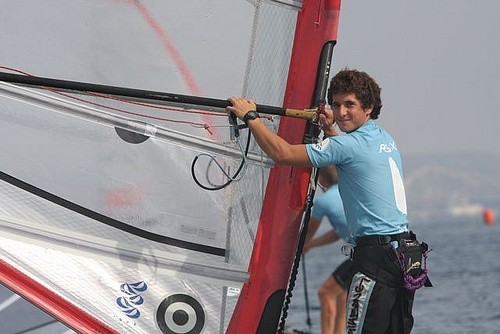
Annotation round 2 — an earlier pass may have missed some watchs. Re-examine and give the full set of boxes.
[242,110,260,124]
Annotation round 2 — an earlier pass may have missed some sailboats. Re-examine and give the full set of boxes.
[1,0,342,334]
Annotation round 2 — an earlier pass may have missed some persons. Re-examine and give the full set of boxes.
[223,67,426,334]
[299,166,359,334]
